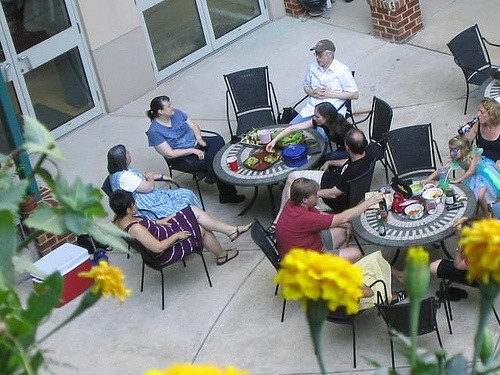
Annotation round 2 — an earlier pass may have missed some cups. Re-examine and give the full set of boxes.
[259,129,271,145]
[227,157,238,171]
[426,200,438,215]
[438,166,448,185]
[380,212,387,223]
[379,185,395,211]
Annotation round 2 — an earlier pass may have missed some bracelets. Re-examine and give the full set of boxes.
[161,173,164,180]
[155,220,157,225]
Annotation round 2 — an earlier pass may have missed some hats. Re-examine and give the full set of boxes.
[310,40,336,52]
[305,139,321,154]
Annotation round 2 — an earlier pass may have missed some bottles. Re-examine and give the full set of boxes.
[458,118,478,135]
[378,191,388,215]
[445,189,455,205]
[376,211,386,236]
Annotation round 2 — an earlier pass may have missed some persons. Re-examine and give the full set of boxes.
[147,96,246,203]
[266,102,369,169]
[431,218,481,289]
[421,135,500,220]
[275,178,384,264]
[110,189,253,266]
[289,40,359,143]
[107,145,204,222]
[457,98,500,168]
[266,129,370,233]
[353,249,406,311]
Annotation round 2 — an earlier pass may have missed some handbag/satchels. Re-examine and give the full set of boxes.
[281,145,307,167]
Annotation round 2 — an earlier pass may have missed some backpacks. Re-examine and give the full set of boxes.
[77,234,113,254]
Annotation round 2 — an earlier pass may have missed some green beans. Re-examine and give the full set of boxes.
[270,130,304,146]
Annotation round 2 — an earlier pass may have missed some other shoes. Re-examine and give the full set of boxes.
[263,223,277,235]
[390,290,408,304]
[220,195,245,203]
[436,286,468,301]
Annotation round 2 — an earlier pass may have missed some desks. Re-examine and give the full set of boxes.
[213,124,326,219]
[352,177,477,265]
[479,76,500,105]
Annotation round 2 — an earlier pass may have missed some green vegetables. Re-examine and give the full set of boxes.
[246,155,279,167]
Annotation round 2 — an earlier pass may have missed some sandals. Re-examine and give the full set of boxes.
[229,223,254,242]
[216,249,239,265]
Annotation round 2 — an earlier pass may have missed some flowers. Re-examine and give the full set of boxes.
[272,218,500,375]
[0,115,249,375]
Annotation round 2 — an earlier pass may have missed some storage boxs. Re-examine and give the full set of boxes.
[29,242,95,308]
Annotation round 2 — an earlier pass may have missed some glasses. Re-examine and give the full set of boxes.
[313,52,326,56]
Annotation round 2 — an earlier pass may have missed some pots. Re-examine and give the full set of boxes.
[281,144,308,167]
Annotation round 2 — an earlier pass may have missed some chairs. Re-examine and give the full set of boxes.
[250,217,286,322]
[145,128,225,212]
[120,236,213,310]
[446,24,500,114]
[374,278,453,371]
[288,71,357,128]
[351,96,394,184]
[224,67,280,141]
[100,175,180,198]
[326,280,388,369]
[384,123,443,180]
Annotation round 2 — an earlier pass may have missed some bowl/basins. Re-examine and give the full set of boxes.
[409,183,425,197]
[422,188,443,204]
[404,204,424,220]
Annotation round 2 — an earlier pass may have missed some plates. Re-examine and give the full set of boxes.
[243,151,281,171]
[425,184,437,189]
